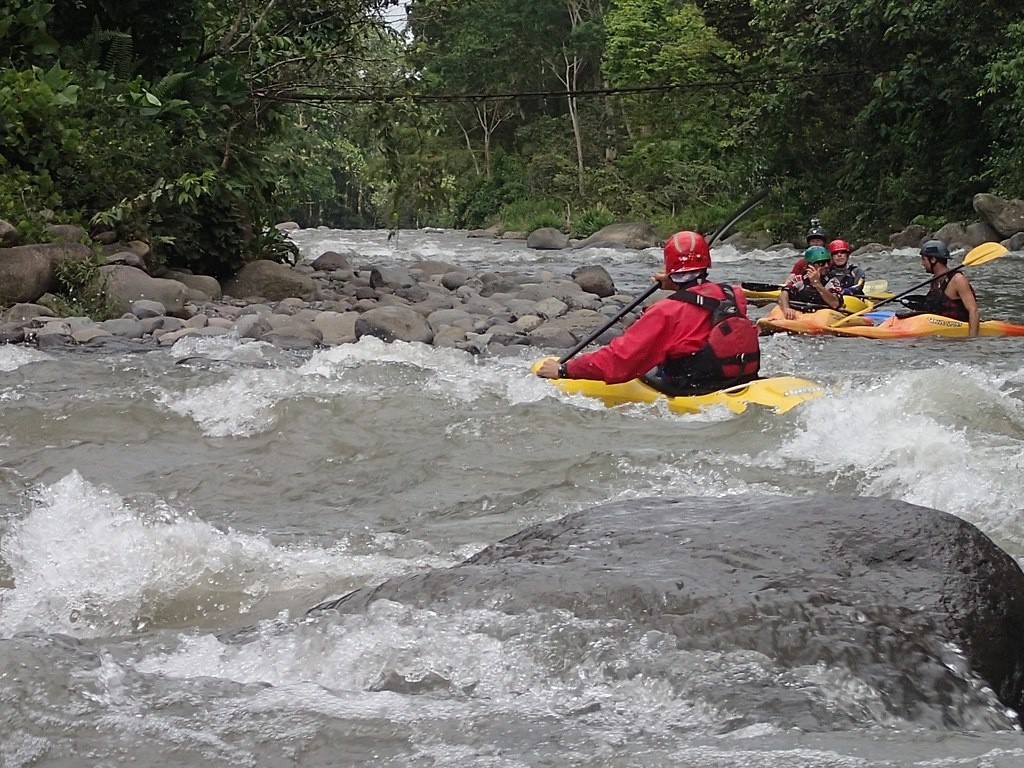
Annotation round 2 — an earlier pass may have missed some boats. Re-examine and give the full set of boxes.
[757,304,848,336]
[532,354,828,419]
[822,311,1024,341]
[740,279,891,299]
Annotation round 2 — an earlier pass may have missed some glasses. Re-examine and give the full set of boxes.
[818,262,825,267]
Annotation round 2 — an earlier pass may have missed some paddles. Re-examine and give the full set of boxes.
[776,278,889,295]
[559,191,771,364]
[740,282,928,312]
[832,242,1007,327]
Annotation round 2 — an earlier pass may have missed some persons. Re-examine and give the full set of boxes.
[780,227,865,320]
[919,240,981,337]
[537,231,760,396]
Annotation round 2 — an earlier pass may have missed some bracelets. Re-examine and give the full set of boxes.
[557,363,567,378]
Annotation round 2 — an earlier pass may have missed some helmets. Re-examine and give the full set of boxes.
[806,227,826,242]
[828,240,849,254]
[664,231,711,277]
[920,239,952,259]
[805,246,831,265]
